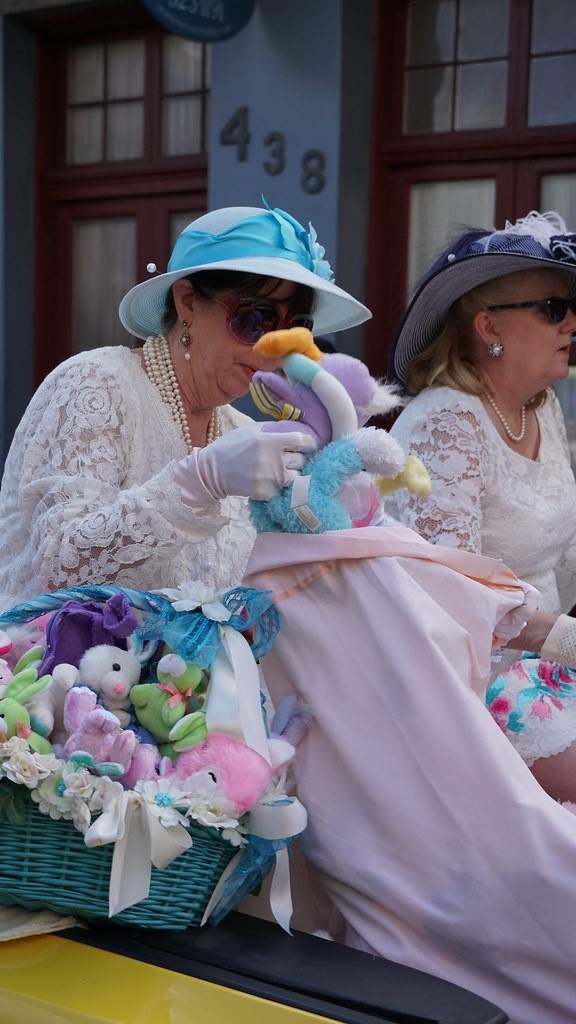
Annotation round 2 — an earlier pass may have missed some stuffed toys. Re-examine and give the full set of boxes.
[245,327,431,541]
[0,620,293,820]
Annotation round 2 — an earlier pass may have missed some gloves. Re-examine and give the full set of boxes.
[173,420,317,507]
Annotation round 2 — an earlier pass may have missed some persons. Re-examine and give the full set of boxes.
[379,210,576,808]
[0,207,372,615]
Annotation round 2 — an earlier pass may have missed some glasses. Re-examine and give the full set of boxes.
[205,289,315,347]
[487,296,576,324]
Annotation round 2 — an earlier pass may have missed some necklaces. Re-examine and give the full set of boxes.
[143,334,222,455]
[482,389,526,441]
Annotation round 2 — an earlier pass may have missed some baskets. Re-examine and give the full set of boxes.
[0,585,244,931]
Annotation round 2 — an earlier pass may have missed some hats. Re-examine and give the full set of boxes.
[117,193,372,341]
[392,210,576,396]
